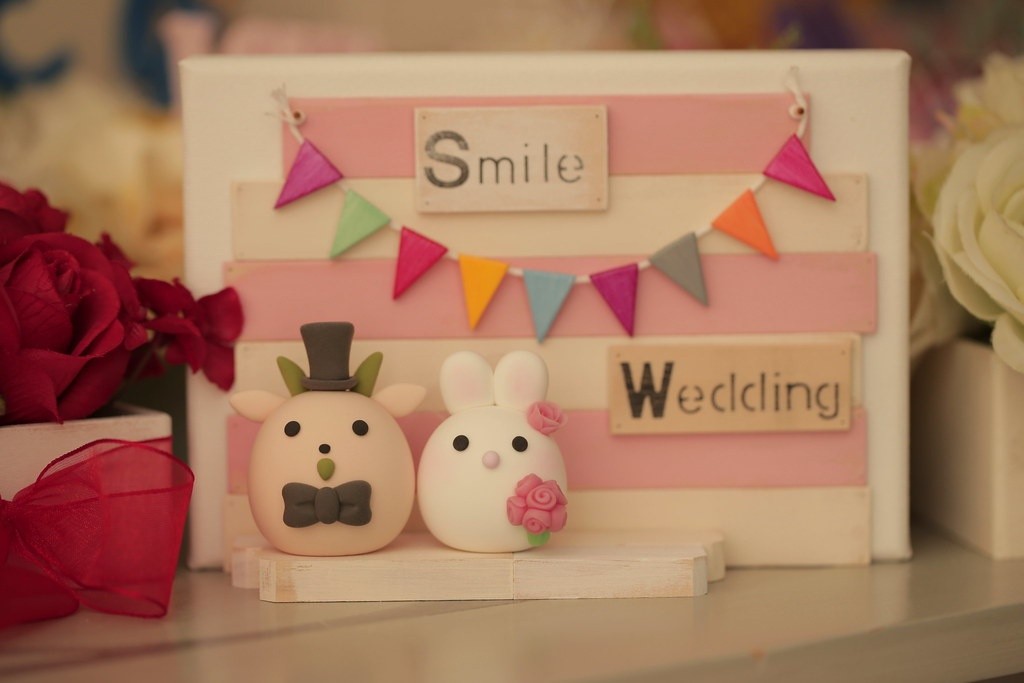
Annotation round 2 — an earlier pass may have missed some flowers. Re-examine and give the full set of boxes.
[905,51,1024,374]
[0,177,243,427]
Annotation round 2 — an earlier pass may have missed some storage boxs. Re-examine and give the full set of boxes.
[908,331,1024,562]
[0,401,173,588]
